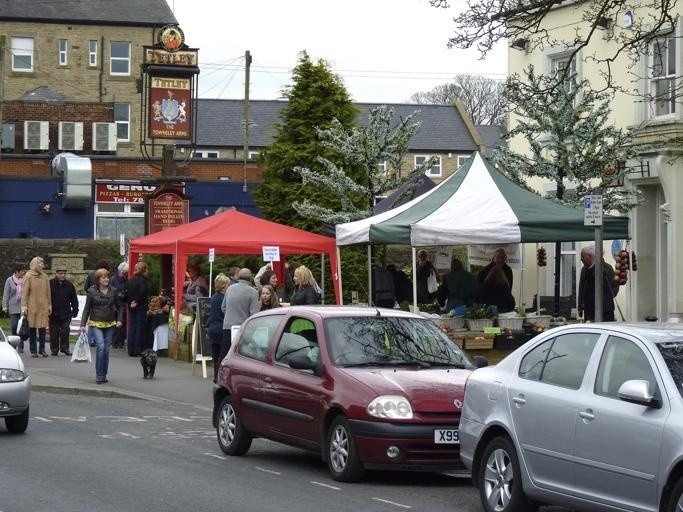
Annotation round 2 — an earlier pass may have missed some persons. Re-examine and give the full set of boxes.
[1,262,26,353]
[576,245,620,325]
[47,267,77,358]
[19,254,52,359]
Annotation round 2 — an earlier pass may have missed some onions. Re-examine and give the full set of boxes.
[613,252,629,287]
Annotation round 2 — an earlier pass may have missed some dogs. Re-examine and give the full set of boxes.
[139,349,158,379]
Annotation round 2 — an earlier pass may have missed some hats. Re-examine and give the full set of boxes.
[56,266,67,272]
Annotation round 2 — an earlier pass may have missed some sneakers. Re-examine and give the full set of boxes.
[96,377,108,384]
[31,349,72,358]
[18,346,24,353]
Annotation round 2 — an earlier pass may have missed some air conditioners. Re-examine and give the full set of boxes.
[58,121,84,151]
[92,121,117,152]
[23,121,51,151]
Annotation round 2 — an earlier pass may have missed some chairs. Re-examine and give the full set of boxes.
[301,329,318,349]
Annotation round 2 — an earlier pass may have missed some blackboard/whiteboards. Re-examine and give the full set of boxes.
[196,297,214,357]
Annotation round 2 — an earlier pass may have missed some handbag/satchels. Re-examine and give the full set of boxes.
[16,315,29,341]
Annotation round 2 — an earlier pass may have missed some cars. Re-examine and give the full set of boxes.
[209,304,488,484]
[454,319,683,512]
[0,326,31,433]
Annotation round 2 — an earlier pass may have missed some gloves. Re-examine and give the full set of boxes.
[71,309,79,318]
